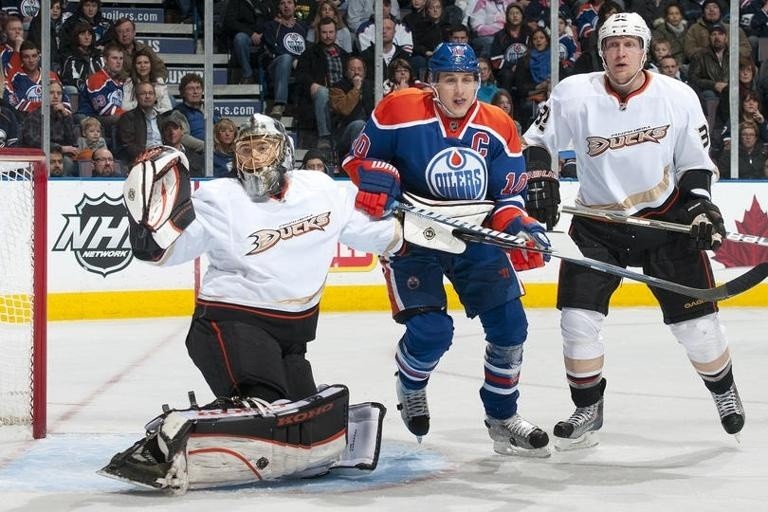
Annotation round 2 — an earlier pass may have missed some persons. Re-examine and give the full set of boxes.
[343,40,554,459]
[96,113,550,491]
[520,12,746,451]
[1,0,768,183]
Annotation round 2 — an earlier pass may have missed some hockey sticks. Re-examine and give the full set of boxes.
[386,196,768,302]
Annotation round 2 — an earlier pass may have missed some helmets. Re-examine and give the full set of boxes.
[597,12,652,57]
[230,114,295,202]
[156,110,190,135]
[426,42,481,83]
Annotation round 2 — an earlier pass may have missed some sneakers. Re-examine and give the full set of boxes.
[554,400,603,439]
[486,414,549,449]
[713,382,745,435]
[394,370,430,435]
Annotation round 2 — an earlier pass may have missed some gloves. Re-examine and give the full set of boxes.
[678,168,726,252]
[490,206,553,272]
[356,158,400,219]
[522,147,562,230]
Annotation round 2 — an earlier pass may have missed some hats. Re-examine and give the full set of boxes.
[710,23,727,34]
